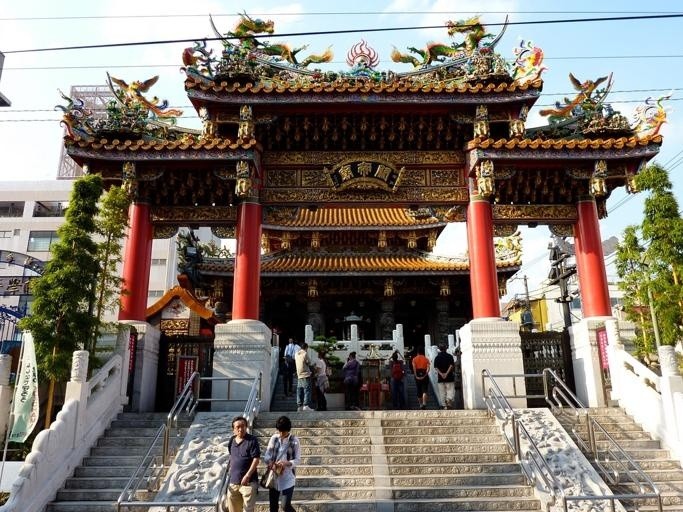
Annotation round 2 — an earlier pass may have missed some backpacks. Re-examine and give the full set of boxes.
[392,361,402,379]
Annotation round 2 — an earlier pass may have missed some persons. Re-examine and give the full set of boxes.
[408,343,417,374]
[264,415,301,512]
[226,416,261,512]
[279,337,328,411]
[411,344,431,408]
[341,352,361,411]
[434,341,455,410]
[388,349,407,409]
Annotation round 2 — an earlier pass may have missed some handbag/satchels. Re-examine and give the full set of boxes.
[260,469,277,489]
[344,376,355,385]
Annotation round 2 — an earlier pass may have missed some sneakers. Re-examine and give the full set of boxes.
[297,406,314,411]
[350,405,361,411]
[444,399,453,410]
